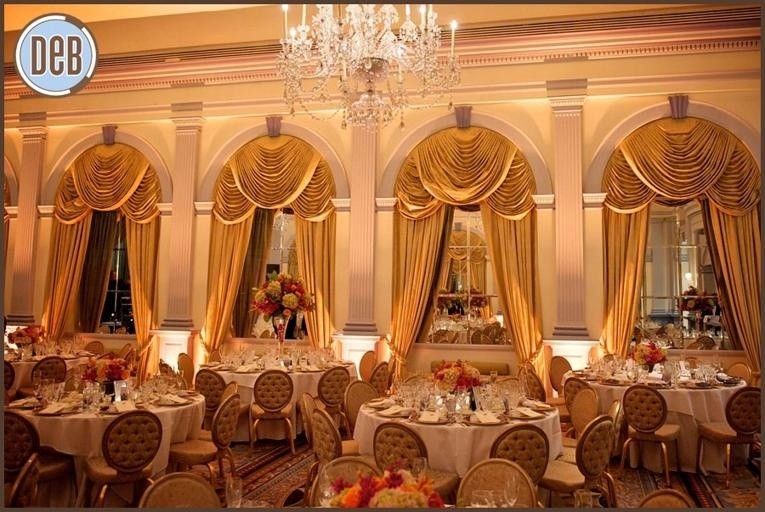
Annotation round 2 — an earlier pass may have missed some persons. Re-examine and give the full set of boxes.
[101,271,125,321]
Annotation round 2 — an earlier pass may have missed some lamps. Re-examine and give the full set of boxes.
[274,3,462,131]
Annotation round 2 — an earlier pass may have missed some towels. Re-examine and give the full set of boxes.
[60,353,76,359]
[235,365,254,373]
[302,365,320,372]
[44,402,68,414]
[160,393,191,404]
[112,399,139,414]
[377,405,405,417]
[513,407,544,419]
[417,410,442,424]
[367,399,395,408]
[523,398,551,410]
[470,411,502,425]
[73,350,93,356]
[264,365,289,372]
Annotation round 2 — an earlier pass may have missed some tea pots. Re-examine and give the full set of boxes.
[211,351,220,362]
[218,344,232,365]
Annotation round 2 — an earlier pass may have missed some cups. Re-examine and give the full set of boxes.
[470,315,497,331]
[633,333,672,349]
[283,359,292,372]
[694,360,705,368]
[392,378,403,405]
[482,380,526,415]
[702,367,716,386]
[223,477,241,510]
[680,362,690,378]
[681,328,729,341]
[83,385,105,413]
[314,487,335,509]
[439,319,466,332]
[132,363,187,410]
[655,365,664,382]
[255,359,264,370]
[31,370,62,412]
[233,342,278,367]
[711,353,720,374]
[670,368,681,390]
[416,368,424,383]
[287,344,334,369]
[504,481,519,508]
[445,399,456,427]
[299,356,307,368]
[410,458,426,493]
[635,367,647,385]
[400,380,446,421]
[317,463,335,493]
[490,371,497,386]
[458,385,467,411]
[471,489,490,509]
[585,354,635,382]
[694,369,704,383]
[573,489,592,509]
[472,387,483,414]
[114,381,128,401]
[679,350,688,361]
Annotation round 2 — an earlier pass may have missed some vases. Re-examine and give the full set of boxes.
[273,315,290,355]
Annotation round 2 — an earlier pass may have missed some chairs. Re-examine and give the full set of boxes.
[317,366,351,444]
[544,415,618,508]
[250,370,298,456]
[639,489,697,510]
[549,356,590,396]
[368,361,389,397]
[170,394,241,485]
[116,343,132,360]
[84,341,105,355]
[299,393,360,483]
[456,458,537,508]
[373,421,458,507]
[490,424,550,485]
[195,369,250,431]
[696,387,761,488]
[559,400,621,463]
[618,385,682,487]
[308,456,382,511]
[564,376,589,405]
[726,362,752,385]
[523,361,566,405]
[77,410,163,509]
[3,411,75,509]
[125,348,136,370]
[344,380,379,439]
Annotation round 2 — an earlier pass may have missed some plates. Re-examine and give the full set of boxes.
[296,369,323,373]
[8,398,42,410]
[229,370,259,374]
[224,498,270,510]
[153,398,192,406]
[376,409,410,418]
[177,390,200,397]
[503,410,545,420]
[409,412,449,424]
[461,416,509,426]
[33,404,81,416]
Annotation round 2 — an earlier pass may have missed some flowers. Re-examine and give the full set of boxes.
[626,342,668,367]
[433,359,483,393]
[316,467,444,508]
[679,290,712,310]
[463,287,487,306]
[82,359,131,381]
[249,270,316,323]
[8,328,38,344]
